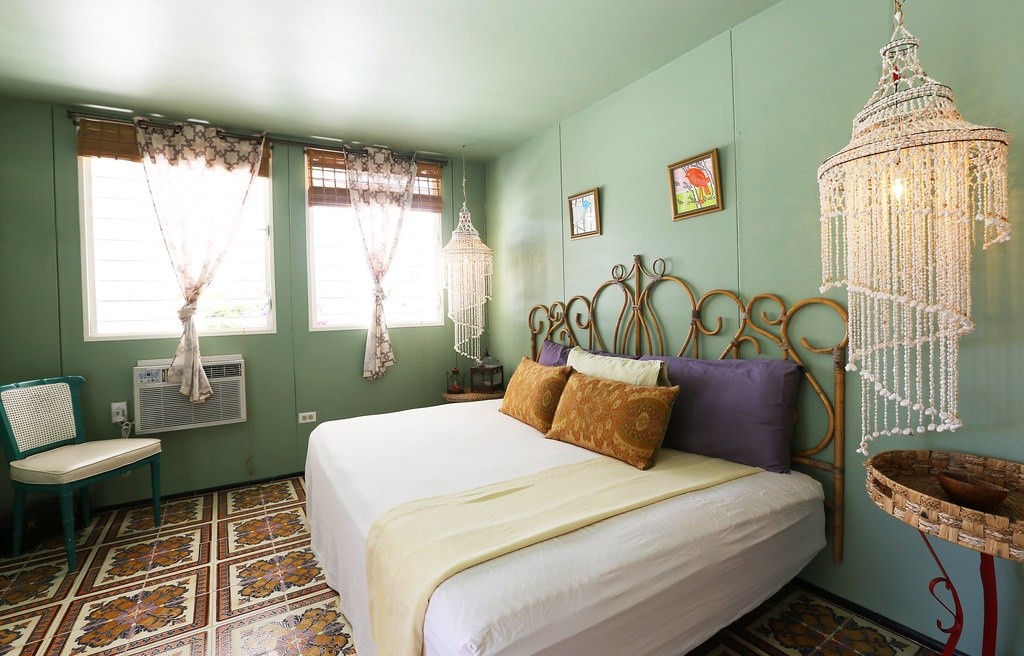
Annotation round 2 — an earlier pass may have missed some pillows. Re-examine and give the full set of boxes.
[498,339,803,475]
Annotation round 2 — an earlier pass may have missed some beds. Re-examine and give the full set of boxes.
[304,255,850,655]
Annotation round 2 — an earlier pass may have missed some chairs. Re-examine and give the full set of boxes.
[0,375,163,573]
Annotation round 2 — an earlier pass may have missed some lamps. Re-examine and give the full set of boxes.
[817,0,1010,456]
[441,145,494,362]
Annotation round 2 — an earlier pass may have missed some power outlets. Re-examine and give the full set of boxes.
[299,412,316,423]
[109,401,128,423]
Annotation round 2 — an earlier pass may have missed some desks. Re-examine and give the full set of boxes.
[442,387,504,402]
[865,450,1024,656]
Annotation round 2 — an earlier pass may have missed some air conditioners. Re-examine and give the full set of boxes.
[132,355,247,434]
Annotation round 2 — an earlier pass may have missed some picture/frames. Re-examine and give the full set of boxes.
[568,187,602,241]
[667,148,724,222]
